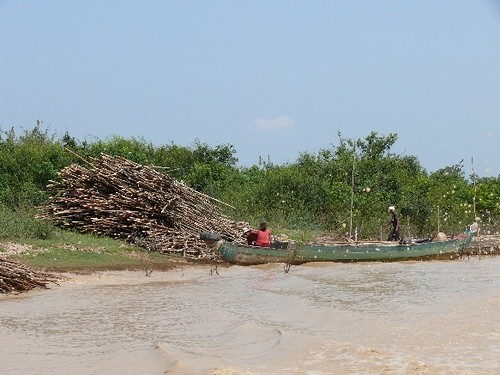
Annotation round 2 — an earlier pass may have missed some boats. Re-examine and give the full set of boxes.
[399,235,500,255]
[199,226,479,264]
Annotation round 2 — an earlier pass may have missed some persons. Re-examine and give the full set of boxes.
[383,206,399,241]
[254,222,271,246]
